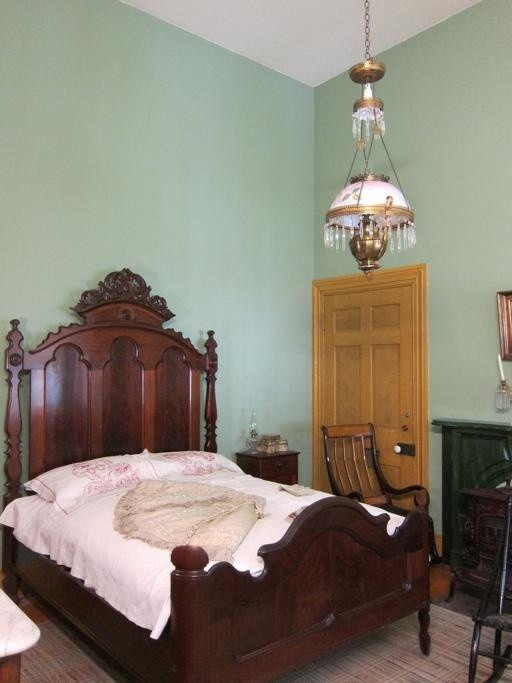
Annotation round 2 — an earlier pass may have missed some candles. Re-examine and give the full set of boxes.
[497,353,505,381]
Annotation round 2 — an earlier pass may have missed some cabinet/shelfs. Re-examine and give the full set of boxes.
[431,416,511,605]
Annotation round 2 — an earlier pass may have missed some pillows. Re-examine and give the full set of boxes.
[18,447,248,515]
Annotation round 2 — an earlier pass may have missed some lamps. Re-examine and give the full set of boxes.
[323,0,417,280]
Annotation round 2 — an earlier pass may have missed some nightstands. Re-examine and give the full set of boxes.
[235,448,301,487]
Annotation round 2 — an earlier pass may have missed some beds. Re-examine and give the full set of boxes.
[6,267,427,683]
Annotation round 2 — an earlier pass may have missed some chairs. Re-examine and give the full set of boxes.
[319,422,431,525]
